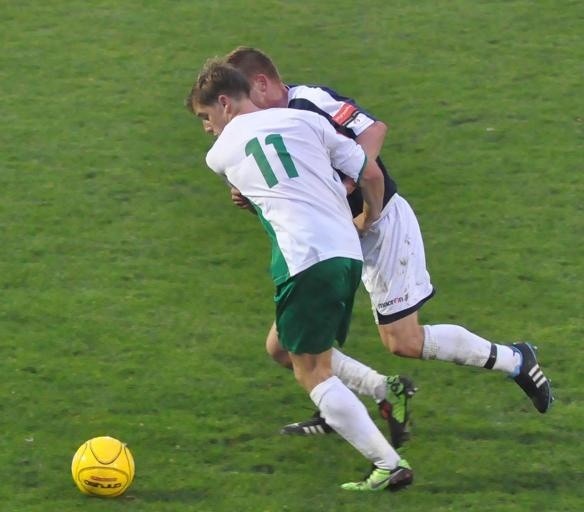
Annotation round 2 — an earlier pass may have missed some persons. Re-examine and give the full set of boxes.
[187,53,416,494]
[221,43,551,437]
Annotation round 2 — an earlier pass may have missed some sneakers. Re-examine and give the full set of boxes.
[281,410,336,436]
[340,374,418,493]
[509,342,553,414]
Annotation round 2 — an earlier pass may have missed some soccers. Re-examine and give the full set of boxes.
[71,436,136,499]
